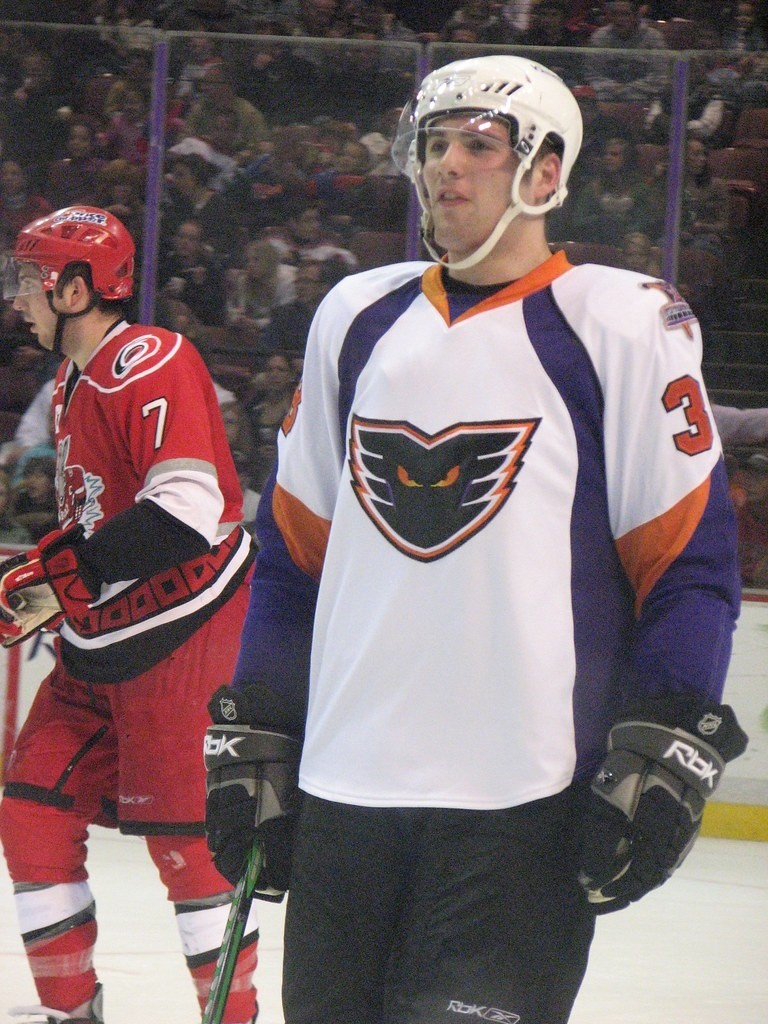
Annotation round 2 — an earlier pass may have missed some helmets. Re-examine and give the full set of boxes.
[409,55,583,215]
[12,204,135,299]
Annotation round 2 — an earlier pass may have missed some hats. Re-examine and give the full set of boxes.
[166,137,210,161]
[747,453,768,473]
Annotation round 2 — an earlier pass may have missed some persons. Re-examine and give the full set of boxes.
[203,52,742,1024]
[0,205,264,1024]
[0,0,767,548]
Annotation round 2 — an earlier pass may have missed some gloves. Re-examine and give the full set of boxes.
[0,523,101,648]
[202,682,307,904]
[578,691,749,914]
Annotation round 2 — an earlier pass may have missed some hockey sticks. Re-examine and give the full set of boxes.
[7,591,64,634]
[201,832,270,1024]
